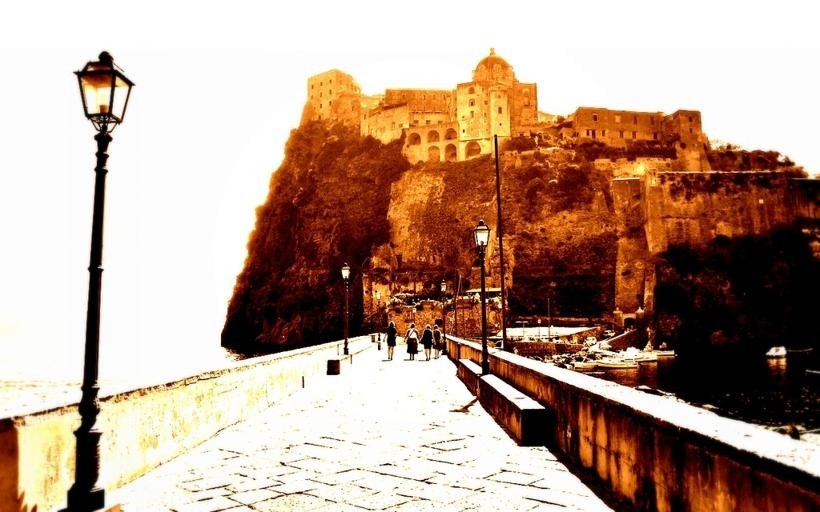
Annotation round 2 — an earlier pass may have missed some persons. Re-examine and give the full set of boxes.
[432,324,442,359]
[382,321,399,361]
[403,322,422,360]
[419,323,436,361]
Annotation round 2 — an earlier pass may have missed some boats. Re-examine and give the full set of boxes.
[766,346,787,358]
[524,341,676,371]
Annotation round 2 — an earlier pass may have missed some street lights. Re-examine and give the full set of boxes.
[412,308,417,323]
[473,219,491,375]
[375,291,381,351]
[66,50,135,512]
[340,262,351,355]
[441,279,447,356]
